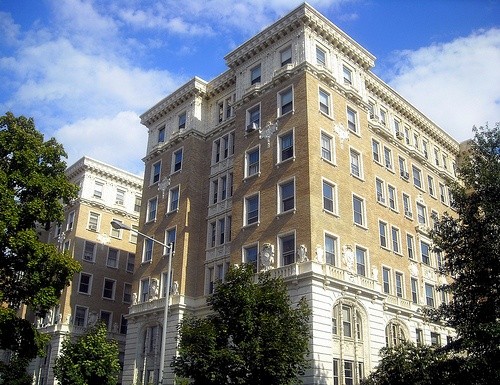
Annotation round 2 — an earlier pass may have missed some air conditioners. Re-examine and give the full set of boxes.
[396,132,404,140]
[247,122,256,132]
[401,171,409,179]
[370,114,379,121]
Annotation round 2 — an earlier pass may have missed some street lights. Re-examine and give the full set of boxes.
[110,220,173,385]
